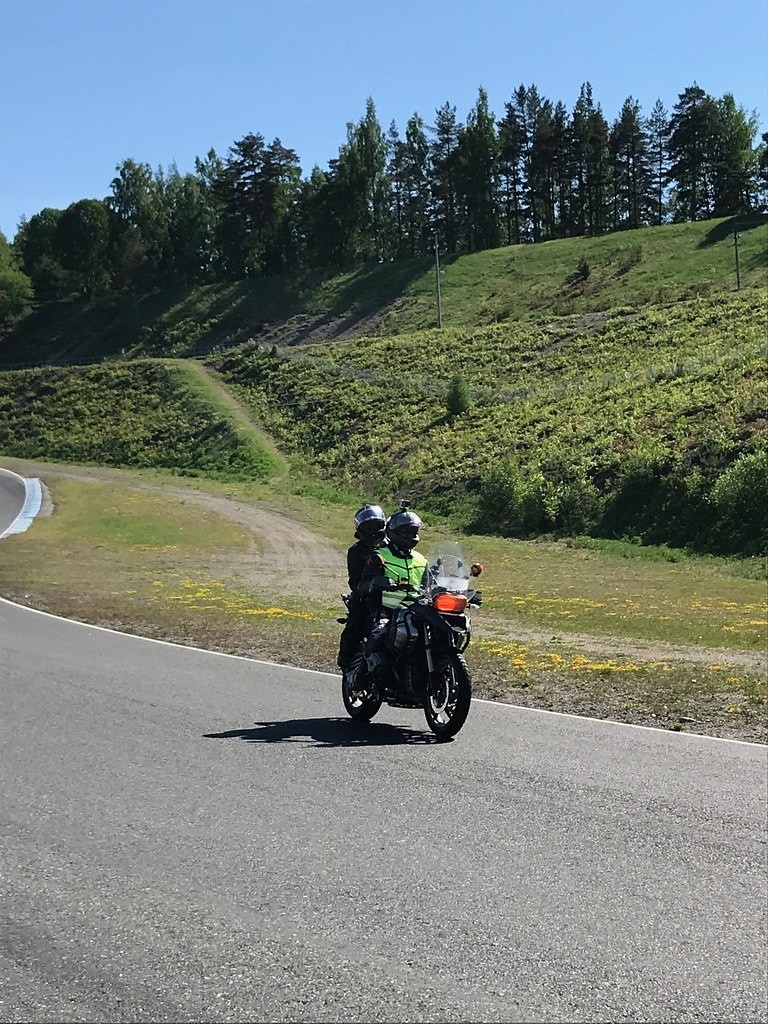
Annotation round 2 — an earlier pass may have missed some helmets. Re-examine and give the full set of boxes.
[386,511,422,549]
[353,507,385,543]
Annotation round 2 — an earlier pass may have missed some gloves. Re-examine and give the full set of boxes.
[372,577,391,592]
[465,589,482,605]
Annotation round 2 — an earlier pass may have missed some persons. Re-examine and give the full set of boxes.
[338,503,483,691]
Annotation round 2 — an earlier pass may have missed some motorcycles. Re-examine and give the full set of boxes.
[337,540,482,737]
